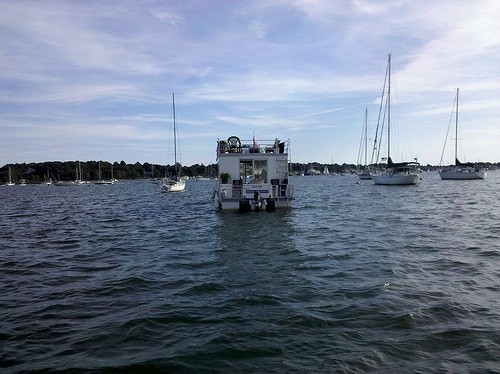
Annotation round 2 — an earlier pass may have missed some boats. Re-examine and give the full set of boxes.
[305,165,321,175]
[209,131,296,214]
[331,173,337,176]
[341,173,345,176]
[137,175,217,184]
[20,180,26,186]
[322,165,330,175]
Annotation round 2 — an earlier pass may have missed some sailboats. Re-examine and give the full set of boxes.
[356,52,421,185]
[42,159,119,187]
[437,88,489,180]
[0,166,15,187]
[158,92,186,193]
